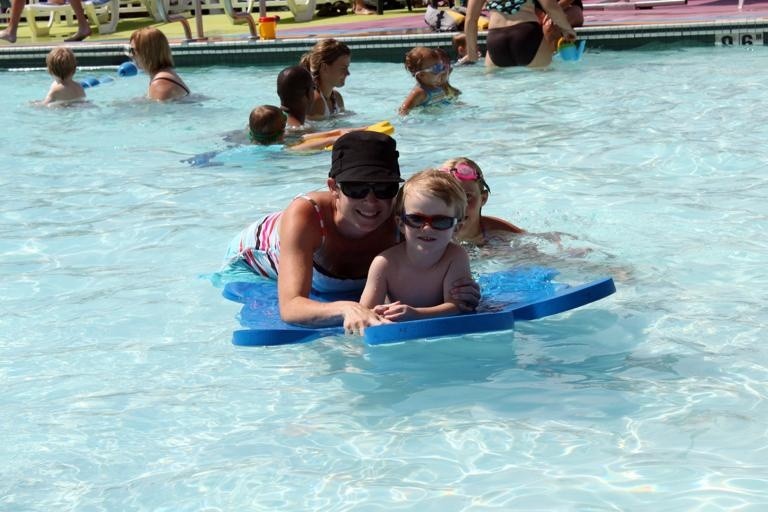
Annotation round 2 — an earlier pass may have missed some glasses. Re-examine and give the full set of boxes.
[414,64,443,78]
[439,162,491,192]
[335,182,399,200]
[401,212,461,230]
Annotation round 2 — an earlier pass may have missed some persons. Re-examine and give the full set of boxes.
[232,130,480,337]
[433,48,461,100]
[454,0,576,68]
[0,0,92,42]
[300,39,351,120]
[277,67,315,134]
[43,47,85,105]
[452,34,481,64]
[353,0,376,15]
[399,47,446,114]
[437,157,523,244]
[535,0,584,51]
[210,104,367,164]
[359,169,471,320]
[130,28,190,102]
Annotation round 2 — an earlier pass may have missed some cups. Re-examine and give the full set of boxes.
[257,16,277,39]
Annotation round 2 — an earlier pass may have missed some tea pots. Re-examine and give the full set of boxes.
[558,36,586,62]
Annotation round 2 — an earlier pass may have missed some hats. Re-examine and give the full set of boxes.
[328,132,405,182]
